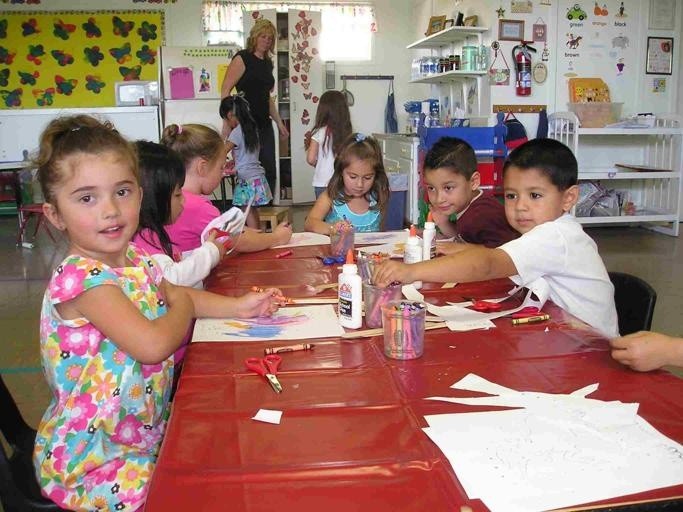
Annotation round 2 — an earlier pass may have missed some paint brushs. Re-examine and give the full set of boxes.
[367,280,394,358]
[276,249,293,257]
[284,222,290,227]
[384,303,425,358]
[264,343,316,354]
[329,214,352,258]
[512,314,549,324]
[357,250,389,279]
[251,286,295,304]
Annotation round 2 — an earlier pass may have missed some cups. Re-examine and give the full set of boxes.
[330,228,354,259]
[381,300,427,361]
[145,95,152,106]
[364,282,403,330]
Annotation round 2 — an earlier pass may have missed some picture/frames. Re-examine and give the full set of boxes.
[648,1,676,30]
[426,15,446,35]
[445,19,454,27]
[490,69,510,86]
[646,37,674,75]
[463,16,477,25]
[498,19,524,42]
[533,24,547,42]
[533,63,547,83]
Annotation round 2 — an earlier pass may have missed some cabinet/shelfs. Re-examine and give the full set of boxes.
[405,26,488,119]
[371,132,417,225]
[158,44,241,200]
[243,8,326,204]
[546,111,681,237]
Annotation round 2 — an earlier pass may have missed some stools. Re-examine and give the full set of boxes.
[254,206,291,228]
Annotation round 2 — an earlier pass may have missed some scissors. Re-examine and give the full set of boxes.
[499,307,545,318]
[315,255,344,264]
[471,296,501,310]
[244,355,283,393]
[208,223,243,248]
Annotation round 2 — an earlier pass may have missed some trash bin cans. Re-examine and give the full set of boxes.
[384,172,408,230]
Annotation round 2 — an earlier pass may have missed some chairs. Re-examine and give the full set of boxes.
[16,204,56,244]
[602,263,657,338]
[0,374,78,511]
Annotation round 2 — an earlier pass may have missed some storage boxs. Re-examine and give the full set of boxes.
[564,101,622,127]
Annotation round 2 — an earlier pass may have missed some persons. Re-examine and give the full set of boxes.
[218,19,289,204]
[30,113,286,508]
[130,140,226,288]
[303,133,391,233]
[369,137,616,339]
[220,95,272,227]
[130,123,293,253]
[609,329,683,373]
[305,90,356,200]
[423,139,522,249]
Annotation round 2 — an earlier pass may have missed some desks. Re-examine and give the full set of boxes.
[0,161,35,229]
[211,173,237,214]
[144,229,683,511]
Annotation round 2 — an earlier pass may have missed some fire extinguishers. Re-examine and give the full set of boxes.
[513,40,535,97]
